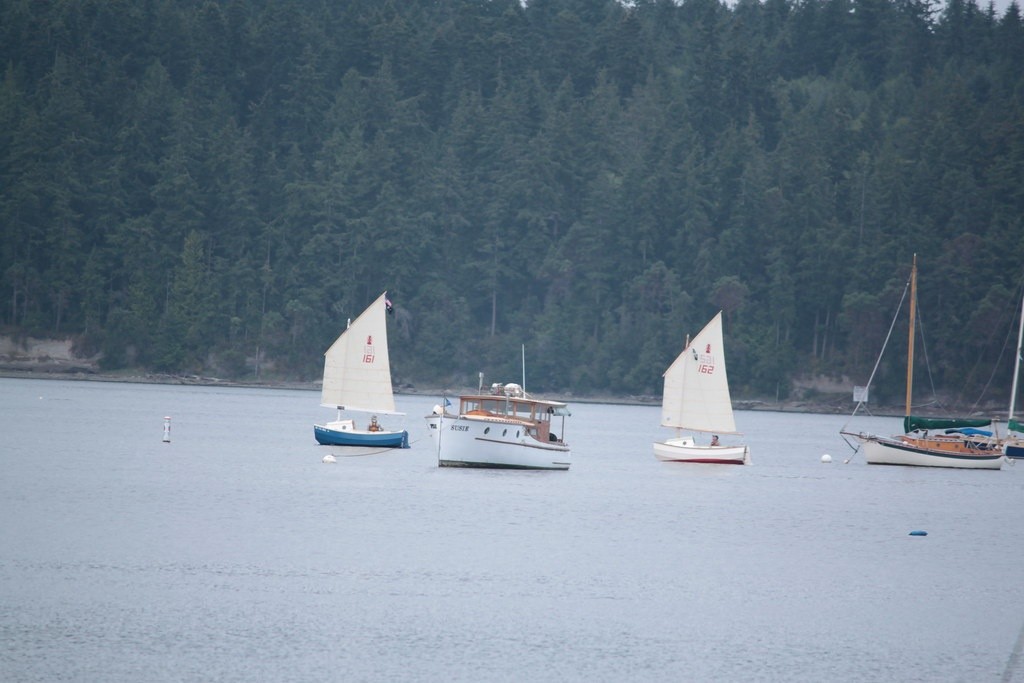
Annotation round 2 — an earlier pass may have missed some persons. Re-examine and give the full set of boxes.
[368,415,385,431]
[711,435,720,446]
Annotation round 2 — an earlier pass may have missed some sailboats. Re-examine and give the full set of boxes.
[313,289,412,450]
[1002,287,1024,459]
[653,309,748,466]
[840,251,1005,470]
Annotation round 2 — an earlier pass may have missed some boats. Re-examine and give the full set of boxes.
[425,344,572,471]
